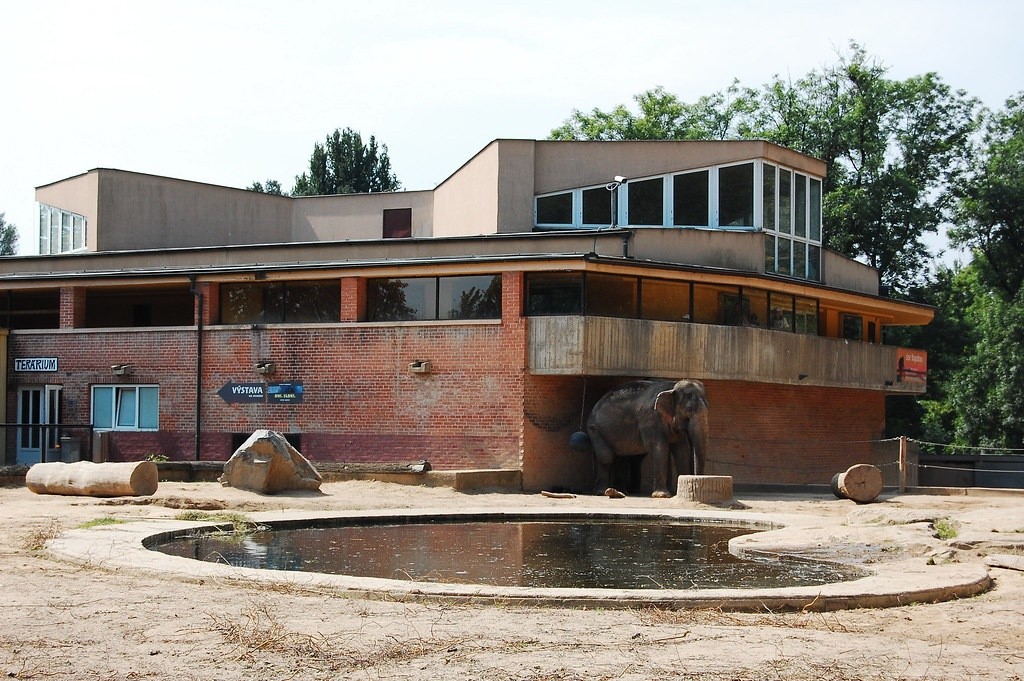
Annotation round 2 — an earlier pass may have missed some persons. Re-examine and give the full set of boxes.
[770,308,790,330]
[736,313,762,327]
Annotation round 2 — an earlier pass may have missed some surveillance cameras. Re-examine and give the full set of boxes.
[614,175,627,183]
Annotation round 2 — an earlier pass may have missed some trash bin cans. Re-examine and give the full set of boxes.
[93,431,110,462]
[61,436,81,462]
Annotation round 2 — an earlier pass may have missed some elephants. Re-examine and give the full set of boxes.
[586,379,709,496]
